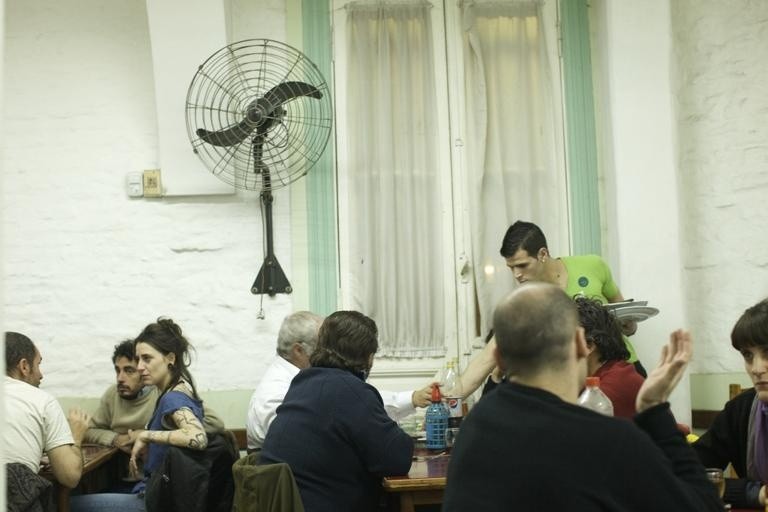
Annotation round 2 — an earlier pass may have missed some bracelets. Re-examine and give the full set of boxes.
[74,440,81,448]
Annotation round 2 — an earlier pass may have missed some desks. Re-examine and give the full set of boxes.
[36,445,120,512]
[382,436,452,512]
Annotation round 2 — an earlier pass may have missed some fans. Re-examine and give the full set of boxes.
[184,37,333,295]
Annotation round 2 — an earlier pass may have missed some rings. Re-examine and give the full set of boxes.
[129,458,132,462]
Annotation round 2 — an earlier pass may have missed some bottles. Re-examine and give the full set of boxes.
[577,377,614,420]
[425,361,464,458]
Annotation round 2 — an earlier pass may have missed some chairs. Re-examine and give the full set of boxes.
[164,429,290,512]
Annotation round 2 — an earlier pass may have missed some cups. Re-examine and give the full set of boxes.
[706,467,726,498]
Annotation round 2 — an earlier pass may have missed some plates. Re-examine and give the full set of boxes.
[603,300,661,323]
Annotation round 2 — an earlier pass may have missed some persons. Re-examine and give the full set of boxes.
[69,319,209,511]
[481,329,507,396]
[421,220,647,431]
[246,311,443,454]
[5,332,92,489]
[83,339,225,493]
[257,310,414,511]
[573,294,646,420]
[689,296,767,511]
[442,291,726,512]
